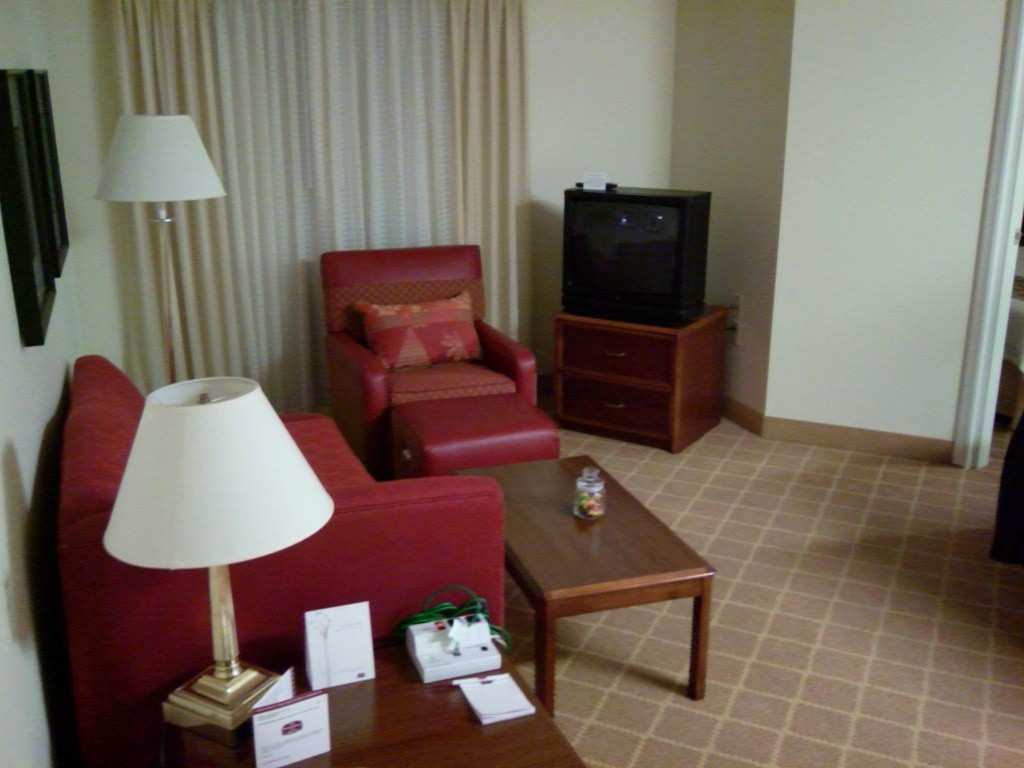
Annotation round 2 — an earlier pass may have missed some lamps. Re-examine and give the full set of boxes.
[93,114,227,382]
[101,377,335,731]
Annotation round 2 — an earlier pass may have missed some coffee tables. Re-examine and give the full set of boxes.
[453,455,717,717]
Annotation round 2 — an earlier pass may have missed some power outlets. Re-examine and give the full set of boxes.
[724,294,743,320]
[725,320,741,345]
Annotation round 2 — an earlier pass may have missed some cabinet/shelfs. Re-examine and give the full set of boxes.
[553,306,725,454]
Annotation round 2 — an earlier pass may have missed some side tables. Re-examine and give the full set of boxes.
[156,646,585,768]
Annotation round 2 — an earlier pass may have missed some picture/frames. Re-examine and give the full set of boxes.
[0,69,57,346]
[28,69,70,277]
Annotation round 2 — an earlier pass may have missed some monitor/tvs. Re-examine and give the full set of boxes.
[560,188,712,328]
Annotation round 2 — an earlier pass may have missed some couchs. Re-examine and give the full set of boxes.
[56,354,506,768]
[317,244,537,471]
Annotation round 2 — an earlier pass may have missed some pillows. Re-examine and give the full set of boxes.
[353,290,481,370]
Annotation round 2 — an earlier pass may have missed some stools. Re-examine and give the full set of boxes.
[390,392,560,477]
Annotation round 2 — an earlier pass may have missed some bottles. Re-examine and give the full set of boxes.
[572,467,606,520]
